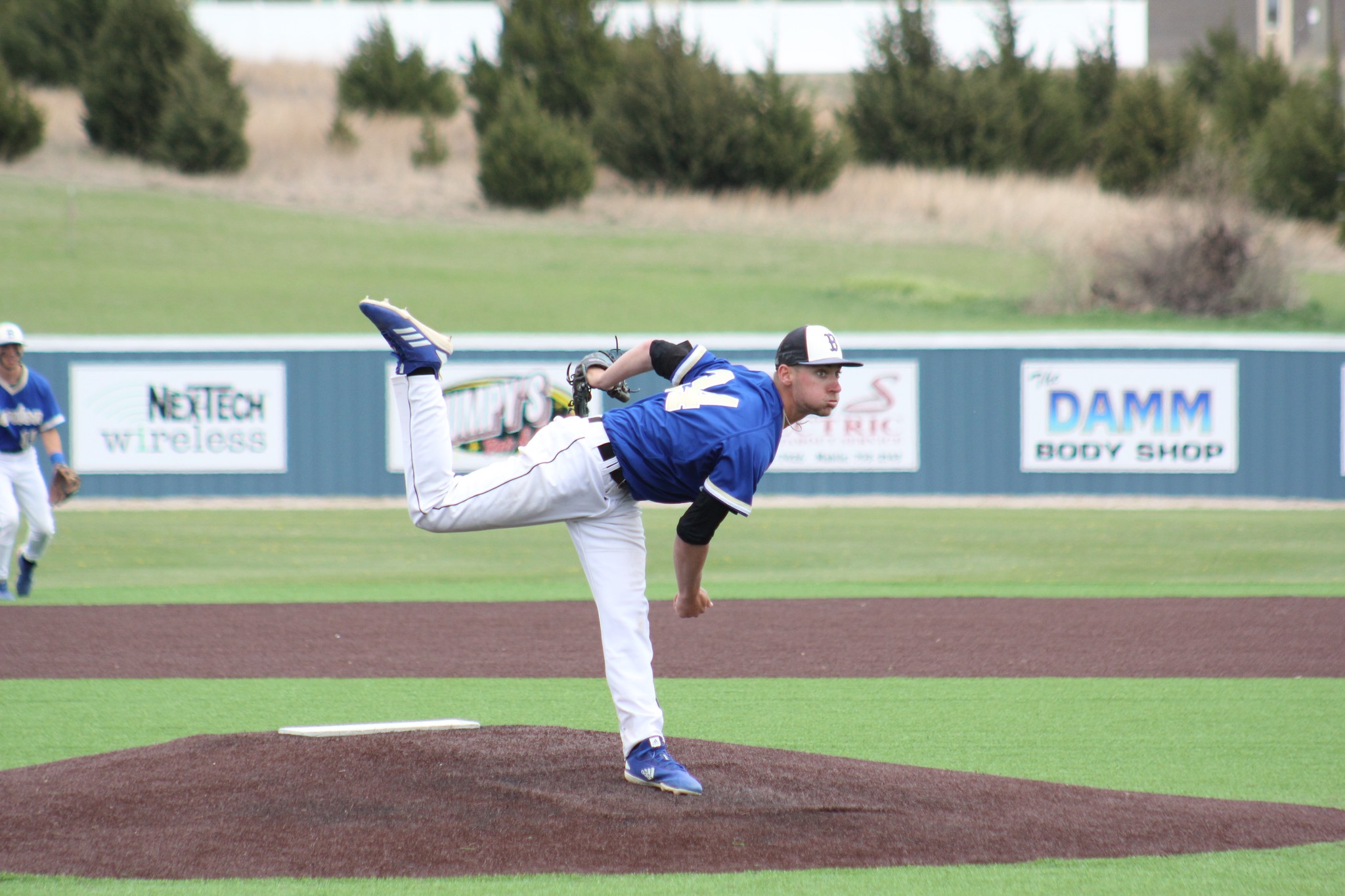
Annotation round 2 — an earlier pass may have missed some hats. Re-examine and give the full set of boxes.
[775,325,864,367]
[0,323,23,346]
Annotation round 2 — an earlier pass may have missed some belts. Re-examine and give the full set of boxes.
[588,417,630,489]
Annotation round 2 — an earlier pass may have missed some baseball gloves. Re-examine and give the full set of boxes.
[572,350,630,418]
[48,466,81,505]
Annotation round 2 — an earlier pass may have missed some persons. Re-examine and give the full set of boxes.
[0,321,81,604]
[357,294,866,798]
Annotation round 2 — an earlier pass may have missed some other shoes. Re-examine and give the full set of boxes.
[16,552,38,598]
[0,580,15,600]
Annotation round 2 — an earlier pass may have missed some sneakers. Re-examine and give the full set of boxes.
[358,295,453,382]
[624,735,703,796]
